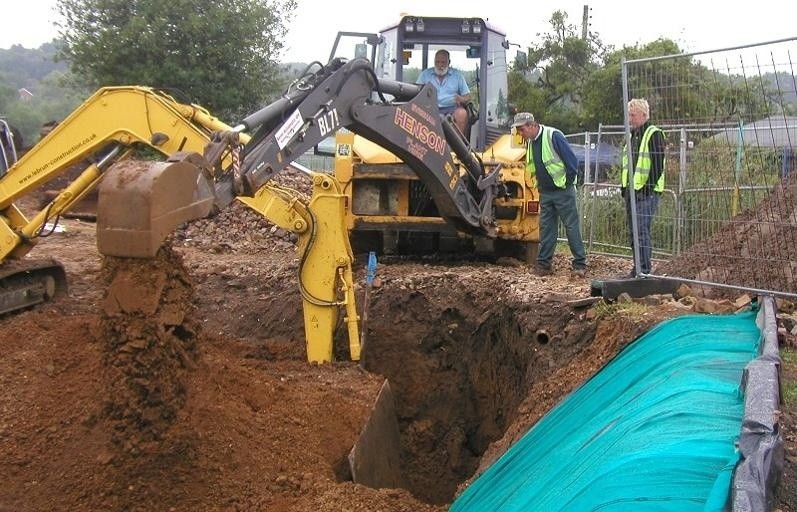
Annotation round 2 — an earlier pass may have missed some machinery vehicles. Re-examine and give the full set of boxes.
[93,12,549,275]
[0,84,366,373]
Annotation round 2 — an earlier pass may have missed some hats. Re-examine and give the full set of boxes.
[511,112,534,127]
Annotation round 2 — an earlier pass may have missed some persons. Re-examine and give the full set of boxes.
[619,96,667,280]
[414,49,474,140]
[508,109,587,275]
